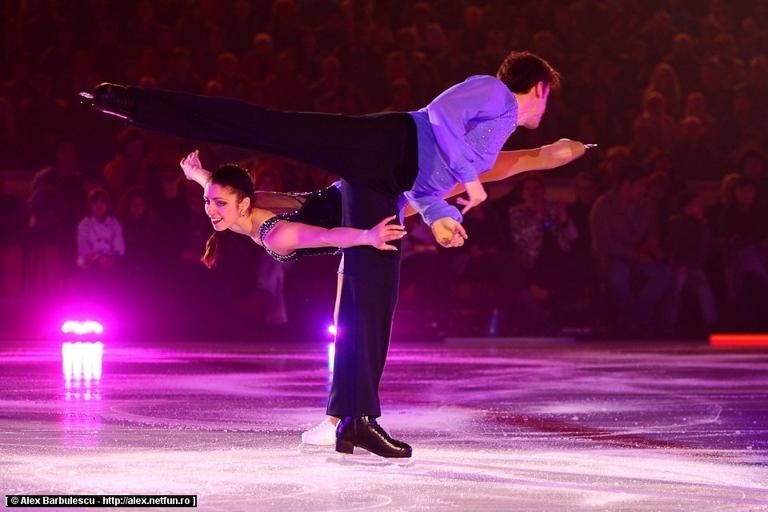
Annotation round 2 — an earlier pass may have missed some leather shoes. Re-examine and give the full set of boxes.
[335,416,413,458]
[91,82,128,119]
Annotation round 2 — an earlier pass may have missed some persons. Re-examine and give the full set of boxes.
[176,136,602,448]
[0,0,767,339]
[52,45,563,460]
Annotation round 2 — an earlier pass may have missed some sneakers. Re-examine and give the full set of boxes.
[300,415,340,446]
